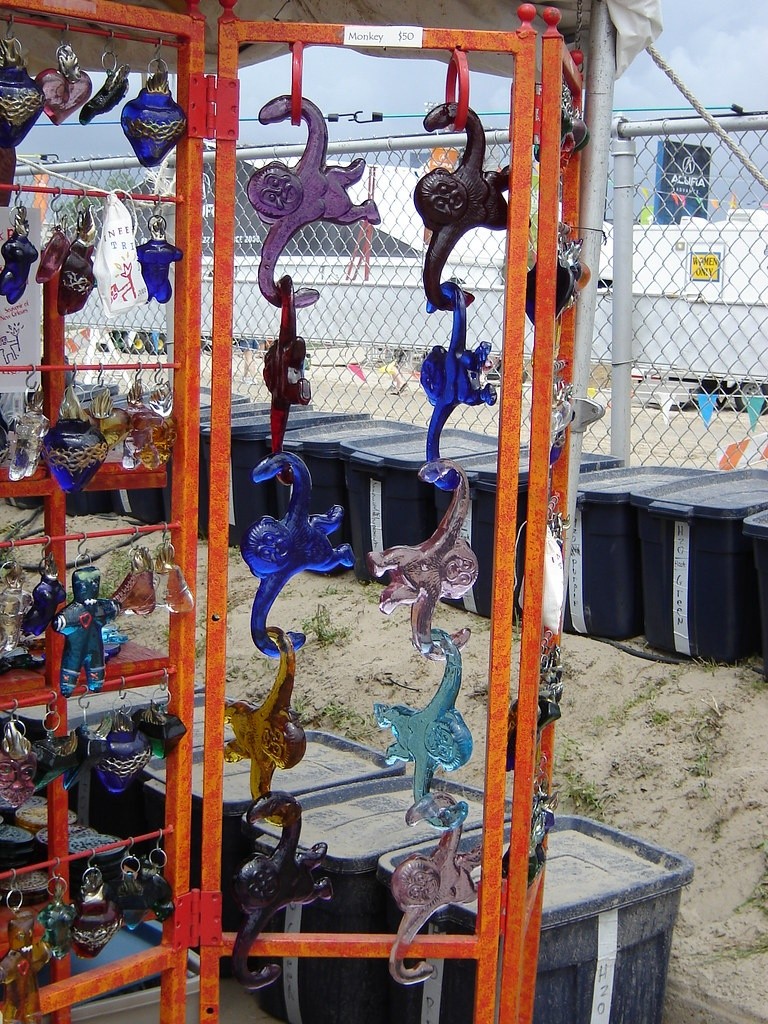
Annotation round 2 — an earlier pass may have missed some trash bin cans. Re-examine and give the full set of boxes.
[265,419,426,577]
[741,509,768,676]
[240,773,513,1024]
[14,684,409,1024]
[375,815,695,1024]
[4,383,370,545]
[337,427,498,586]
[562,465,721,642]
[630,469,768,666]
[433,447,625,619]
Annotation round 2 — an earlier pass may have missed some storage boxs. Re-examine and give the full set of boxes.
[66,390,156,518]
[66,692,259,855]
[142,729,407,980]
[434,448,625,618]
[236,774,513,1024]
[338,427,529,587]
[161,403,313,538]
[629,467,768,663]
[4,682,205,797]
[200,412,370,545]
[741,508,768,679]
[374,813,695,1024]
[548,464,723,639]
[5,383,119,510]
[109,386,252,525]
[264,418,429,578]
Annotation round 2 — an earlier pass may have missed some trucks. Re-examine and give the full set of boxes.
[633,368,767,416]
